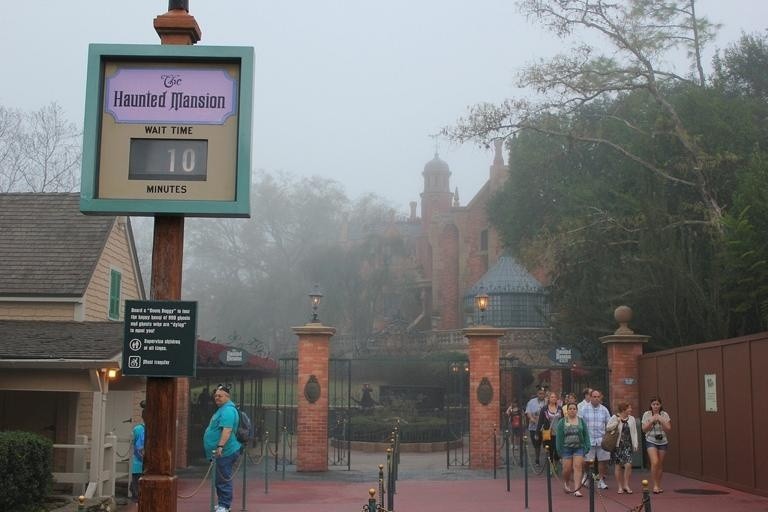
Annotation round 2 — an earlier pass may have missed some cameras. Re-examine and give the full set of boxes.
[655,434,663,440]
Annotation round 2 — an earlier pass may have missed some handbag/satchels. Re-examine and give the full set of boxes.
[601,432,619,453]
[542,430,551,442]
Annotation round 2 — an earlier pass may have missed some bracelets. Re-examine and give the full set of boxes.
[217,444,224,449]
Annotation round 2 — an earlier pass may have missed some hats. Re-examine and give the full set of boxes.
[217,384,229,394]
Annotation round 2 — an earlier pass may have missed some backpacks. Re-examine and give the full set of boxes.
[229,404,251,445]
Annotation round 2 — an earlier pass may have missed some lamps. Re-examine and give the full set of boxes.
[473,286,490,325]
[107,369,115,380]
[307,282,325,323]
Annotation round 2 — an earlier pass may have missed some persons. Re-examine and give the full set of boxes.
[202,385,243,512]
[505,384,672,497]
[129,409,145,500]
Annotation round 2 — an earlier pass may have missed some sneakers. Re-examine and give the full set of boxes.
[598,480,609,489]
[215,504,230,512]
[573,491,582,497]
